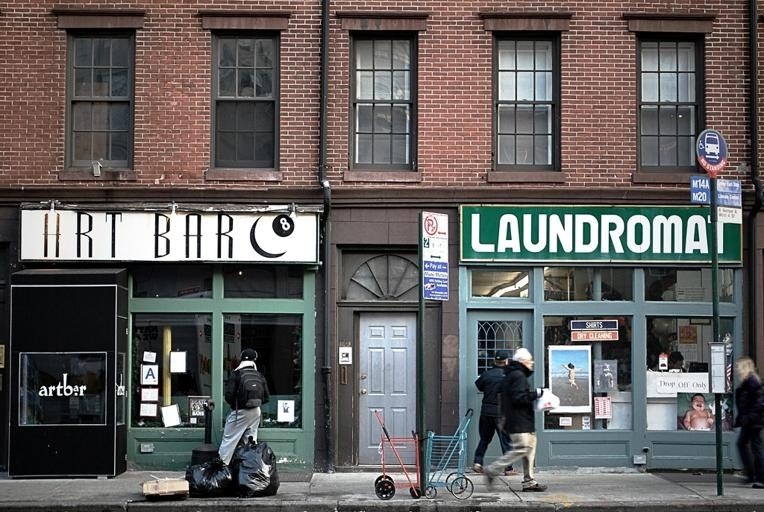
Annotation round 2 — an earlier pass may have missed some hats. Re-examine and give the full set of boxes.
[240,349,258,362]
[495,350,510,360]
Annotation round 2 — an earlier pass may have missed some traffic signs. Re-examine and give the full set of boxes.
[424,261,449,272]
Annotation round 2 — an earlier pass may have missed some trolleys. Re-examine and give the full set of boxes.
[375,408,475,500]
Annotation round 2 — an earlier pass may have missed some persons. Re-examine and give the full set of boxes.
[474,351,521,475]
[726,358,763,488]
[683,394,715,431]
[660,350,688,372]
[595,362,613,390]
[217,348,261,467]
[485,347,547,492]
[561,362,582,390]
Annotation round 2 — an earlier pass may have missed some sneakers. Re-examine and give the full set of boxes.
[505,471,519,476]
[523,482,547,492]
[472,465,484,473]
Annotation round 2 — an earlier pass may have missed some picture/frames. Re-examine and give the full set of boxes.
[549,345,591,413]
[188,396,211,418]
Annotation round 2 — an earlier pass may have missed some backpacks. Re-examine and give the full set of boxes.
[234,369,265,408]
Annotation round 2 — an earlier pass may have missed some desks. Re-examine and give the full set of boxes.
[606,392,678,431]
[158,395,301,418]
